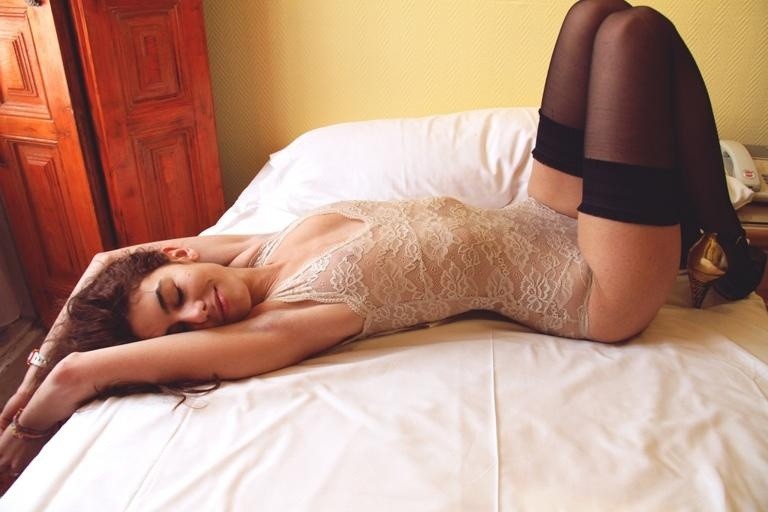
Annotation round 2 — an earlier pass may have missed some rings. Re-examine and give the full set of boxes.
[9,472,18,477]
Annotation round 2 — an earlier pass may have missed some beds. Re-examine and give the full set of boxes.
[0,165,768,512]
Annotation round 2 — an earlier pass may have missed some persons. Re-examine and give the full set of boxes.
[1,0,768,484]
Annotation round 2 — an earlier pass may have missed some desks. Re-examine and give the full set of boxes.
[730,204,767,296]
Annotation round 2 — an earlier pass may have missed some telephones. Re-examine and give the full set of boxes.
[720,141,768,202]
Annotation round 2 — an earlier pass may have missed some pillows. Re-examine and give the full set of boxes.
[270,110,540,209]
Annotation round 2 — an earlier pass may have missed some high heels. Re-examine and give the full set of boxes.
[678,216,766,309]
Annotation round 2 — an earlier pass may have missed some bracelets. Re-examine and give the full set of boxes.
[11,408,59,436]
[25,349,56,372]
[9,423,49,439]
